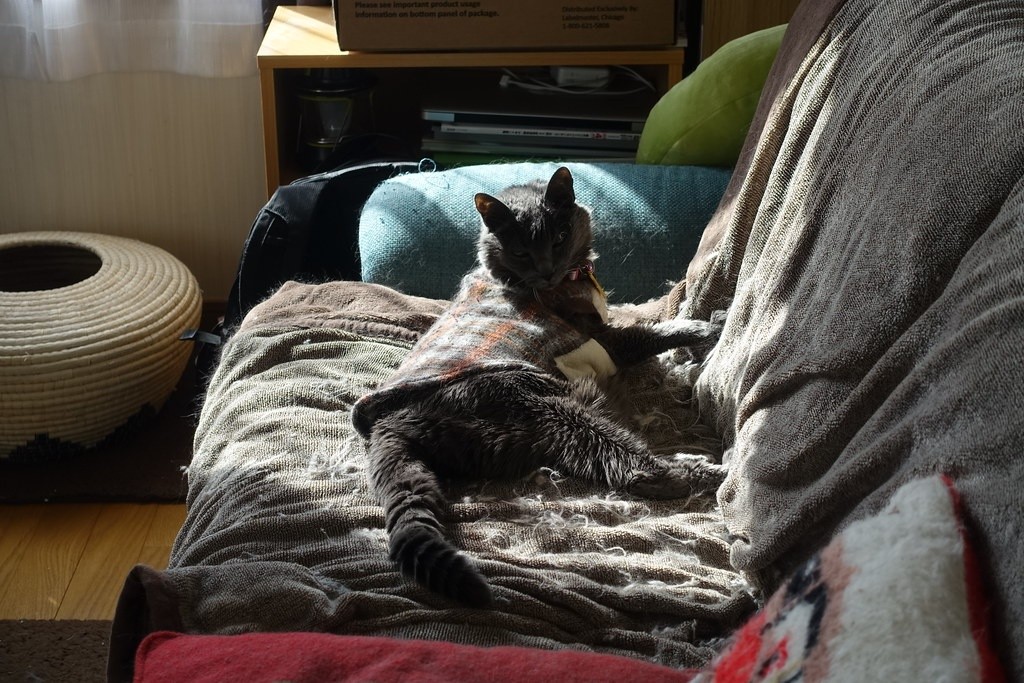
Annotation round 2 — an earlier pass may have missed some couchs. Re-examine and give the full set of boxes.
[107,0,1024,683]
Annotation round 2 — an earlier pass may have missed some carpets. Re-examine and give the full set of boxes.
[0,619,113,683]
[0,303,228,504]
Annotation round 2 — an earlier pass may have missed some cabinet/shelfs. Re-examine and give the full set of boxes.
[256,6,685,201]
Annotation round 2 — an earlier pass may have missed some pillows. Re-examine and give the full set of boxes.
[635,24,787,166]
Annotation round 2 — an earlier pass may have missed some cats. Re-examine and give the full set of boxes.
[366,167,723,611]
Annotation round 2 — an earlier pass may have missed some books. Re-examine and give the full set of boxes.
[420,108,648,164]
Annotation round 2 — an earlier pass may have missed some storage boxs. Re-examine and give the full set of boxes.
[333,0,677,52]
